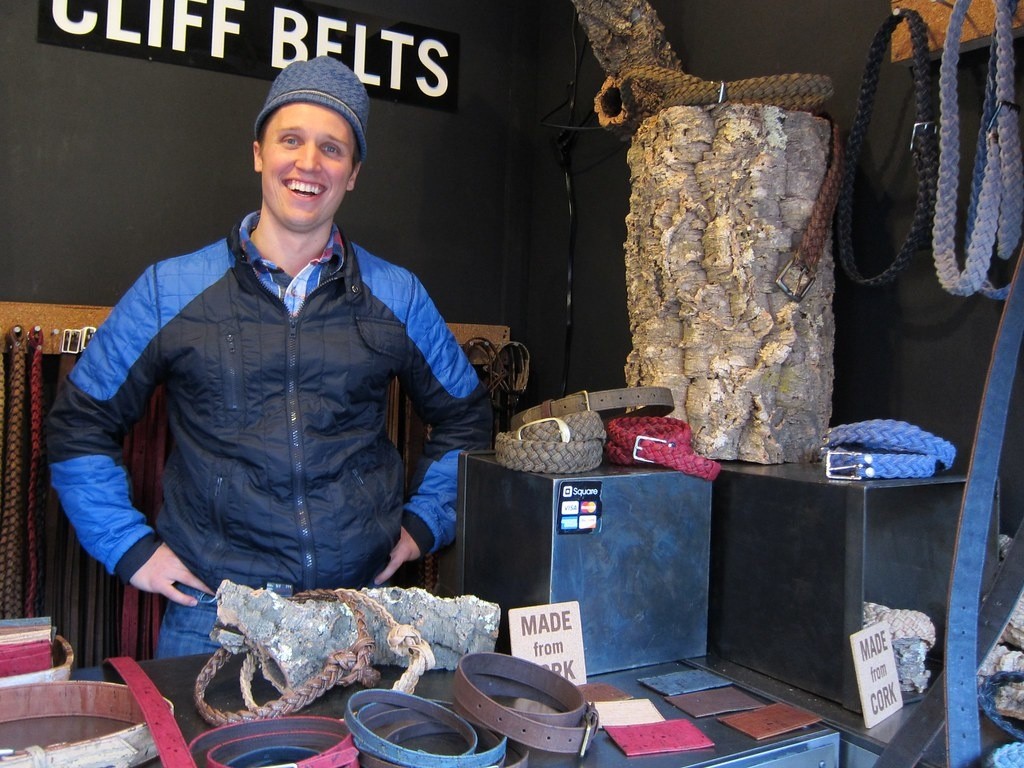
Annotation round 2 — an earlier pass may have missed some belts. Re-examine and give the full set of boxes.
[0,327,530,667]
[496,0,1023,768]
[0,640,600,768]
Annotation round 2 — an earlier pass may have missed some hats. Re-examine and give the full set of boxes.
[253,55,369,162]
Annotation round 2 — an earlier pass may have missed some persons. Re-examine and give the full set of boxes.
[44,56,494,659]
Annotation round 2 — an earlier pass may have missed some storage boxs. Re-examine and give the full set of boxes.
[711,459,1001,712]
[457,450,710,677]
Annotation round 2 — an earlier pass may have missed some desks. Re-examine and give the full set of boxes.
[0,651,1007,768]
